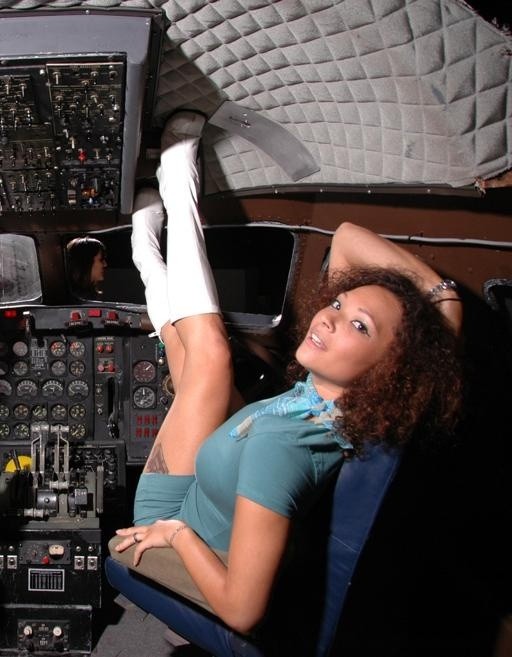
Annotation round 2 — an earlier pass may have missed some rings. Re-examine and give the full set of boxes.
[133,533,141,544]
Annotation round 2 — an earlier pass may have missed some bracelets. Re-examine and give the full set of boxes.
[433,296,463,307]
[168,525,189,547]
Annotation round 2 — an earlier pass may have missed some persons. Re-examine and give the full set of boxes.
[114,104,465,637]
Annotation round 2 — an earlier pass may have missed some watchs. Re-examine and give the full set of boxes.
[425,279,457,301]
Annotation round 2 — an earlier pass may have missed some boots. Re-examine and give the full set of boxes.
[158,109,223,325]
[131,186,170,343]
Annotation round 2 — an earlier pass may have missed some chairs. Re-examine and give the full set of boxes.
[103,437,401,657]
